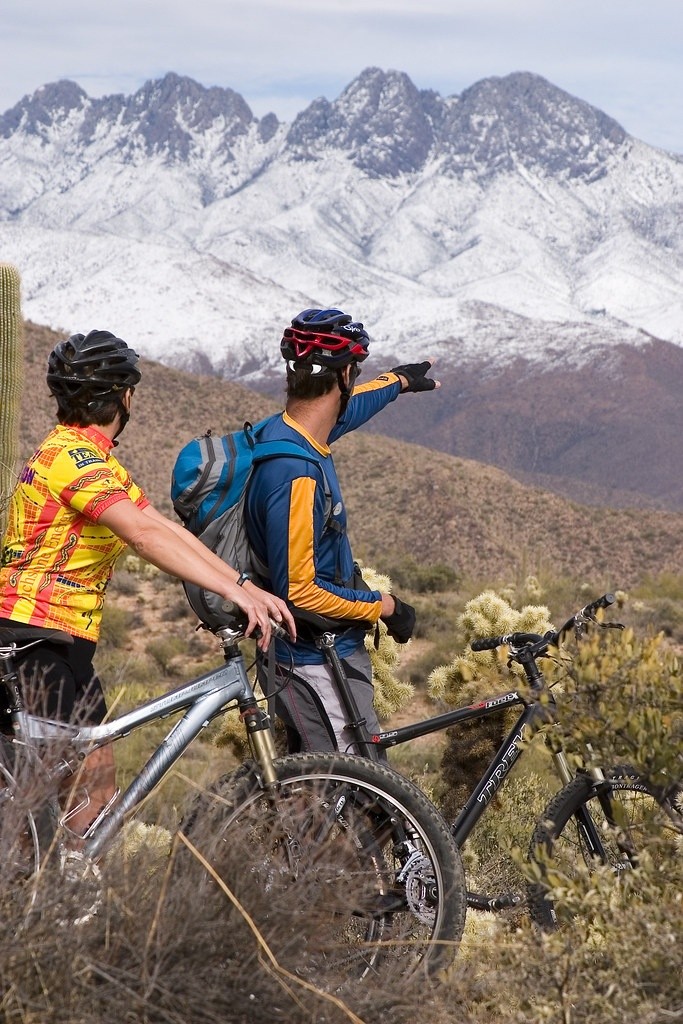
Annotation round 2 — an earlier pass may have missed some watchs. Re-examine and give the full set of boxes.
[236,572,253,585]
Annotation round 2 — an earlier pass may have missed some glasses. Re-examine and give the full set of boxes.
[356,366,361,377]
[123,385,135,397]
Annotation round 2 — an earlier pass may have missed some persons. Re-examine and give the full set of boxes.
[242,307,444,850]
[0,329,297,869]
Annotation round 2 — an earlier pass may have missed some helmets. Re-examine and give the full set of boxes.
[46,329,142,413]
[280,309,370,378]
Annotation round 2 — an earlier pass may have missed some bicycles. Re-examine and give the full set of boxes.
[169,591,683,953]
[0,605,471,1024]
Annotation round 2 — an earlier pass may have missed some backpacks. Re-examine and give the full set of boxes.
[170,412,379,651]
